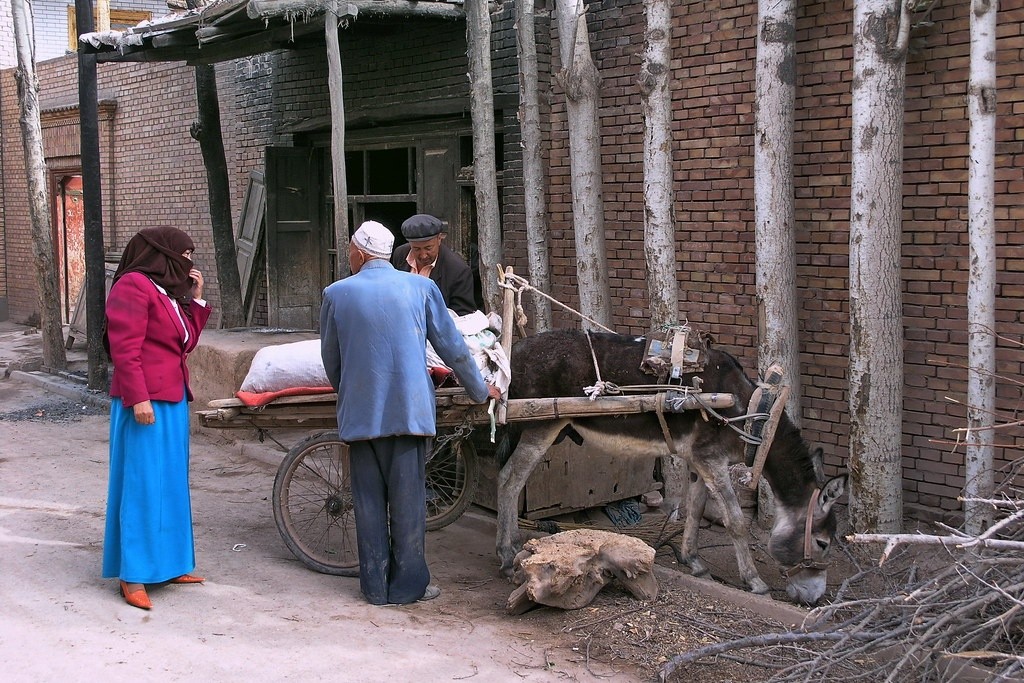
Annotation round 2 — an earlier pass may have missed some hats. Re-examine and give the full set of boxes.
[401,214,443,243]
[351,220,395,259]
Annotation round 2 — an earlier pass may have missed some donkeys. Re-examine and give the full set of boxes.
[495,329,850,606]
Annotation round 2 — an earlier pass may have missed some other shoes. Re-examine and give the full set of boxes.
[420,586,441,601]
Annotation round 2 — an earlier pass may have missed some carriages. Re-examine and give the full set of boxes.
[197,260,849,606]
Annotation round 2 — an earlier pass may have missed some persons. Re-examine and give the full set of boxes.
[390,213,477,501]
[320,219,503,604]
[104,225,213,609]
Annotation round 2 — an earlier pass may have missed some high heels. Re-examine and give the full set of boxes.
[170,574,204,583]
[120,580,153,609]
